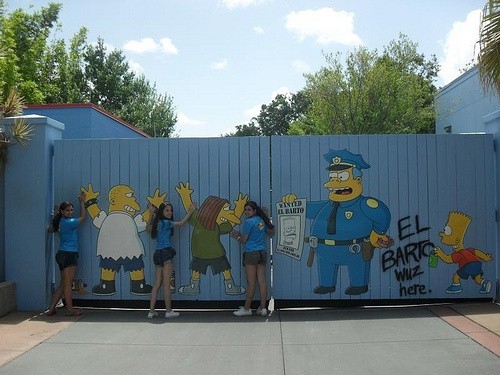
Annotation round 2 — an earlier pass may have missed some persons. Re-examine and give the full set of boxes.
[230,201,275,316]
[147,201,197,318]
[46,192,86,316]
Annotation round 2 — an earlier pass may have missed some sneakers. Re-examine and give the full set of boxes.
[147,311,159,319]
[165,311,180,318]
[233,306,253,317]
[255,306,269,317]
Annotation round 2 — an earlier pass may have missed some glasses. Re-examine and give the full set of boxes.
[244,208,253,211]
[64,208,74,212]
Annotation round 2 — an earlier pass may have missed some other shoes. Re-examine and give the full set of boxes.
[65,308,84,316]
[46,309,58,316]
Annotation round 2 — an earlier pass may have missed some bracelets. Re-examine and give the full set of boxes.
[237,236,241,240]
